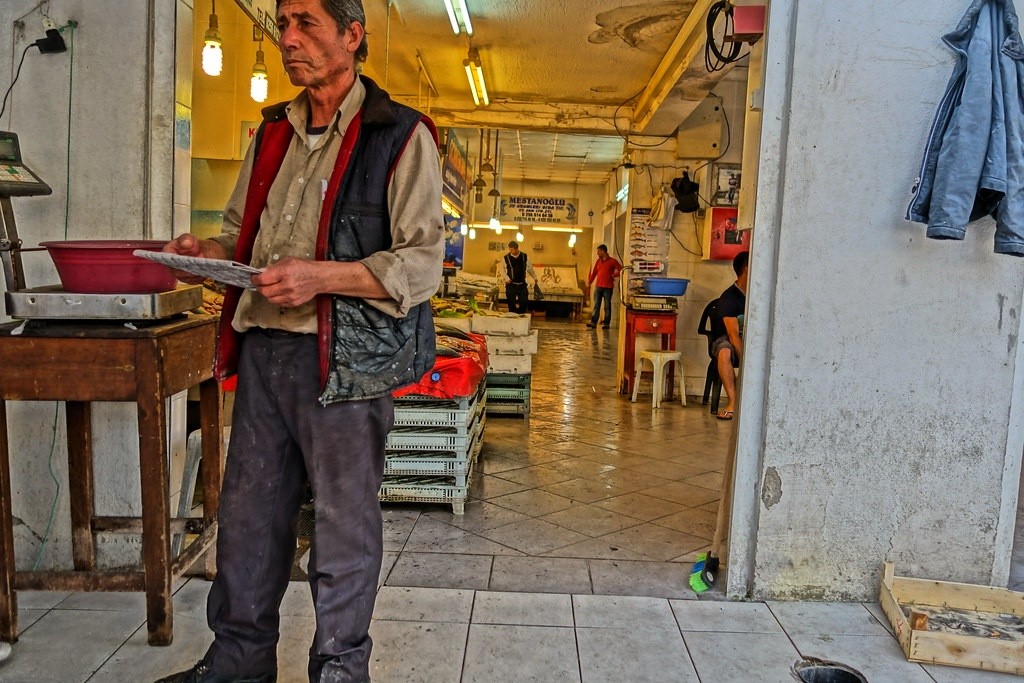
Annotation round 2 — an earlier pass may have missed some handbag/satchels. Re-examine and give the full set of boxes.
[534,283,544,299]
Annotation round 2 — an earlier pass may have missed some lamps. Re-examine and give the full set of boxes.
[615,184,629,201]
[461,219,579,249]
[462,48,493,107]
[443,0,475,35]
[473,128,500,203]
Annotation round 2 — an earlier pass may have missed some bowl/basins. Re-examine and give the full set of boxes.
[643,278,691,295]
[39,240,179,293]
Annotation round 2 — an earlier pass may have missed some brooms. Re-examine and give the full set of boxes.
[686,340,749,597]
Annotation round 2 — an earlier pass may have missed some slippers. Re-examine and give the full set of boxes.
[717,411,733,420]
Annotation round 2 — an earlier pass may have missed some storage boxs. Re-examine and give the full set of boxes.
[189,276,585,516]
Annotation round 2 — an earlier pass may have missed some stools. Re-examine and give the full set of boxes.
[632,349,687,408]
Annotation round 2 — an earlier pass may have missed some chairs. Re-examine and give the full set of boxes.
[699,296,741,415]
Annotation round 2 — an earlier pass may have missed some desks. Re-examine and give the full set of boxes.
[619,308,678,403]
[0,315,230,647]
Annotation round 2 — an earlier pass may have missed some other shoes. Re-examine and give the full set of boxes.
[154,659,277,683]
[586,322,596,328]
[602,325,609,328]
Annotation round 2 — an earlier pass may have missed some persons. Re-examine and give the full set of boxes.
[727,173,738,204]
[585,244,623,330]
[151,0,444,683]
[709,251,749,423]
[498,240,538,315]
